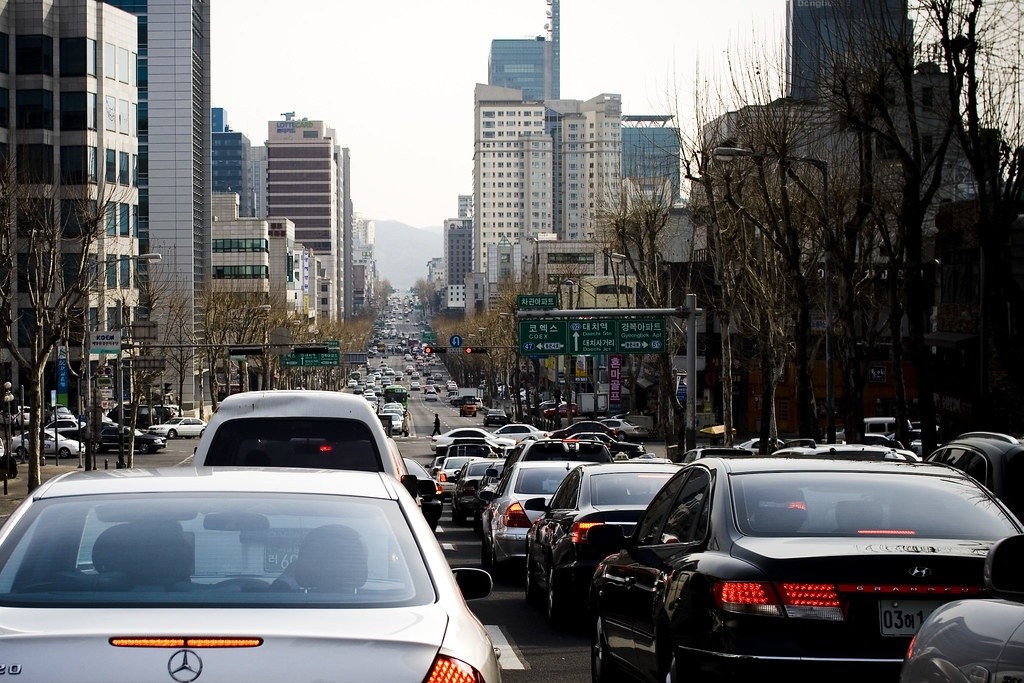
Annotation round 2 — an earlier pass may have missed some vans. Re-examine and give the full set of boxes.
[193,389,401,482]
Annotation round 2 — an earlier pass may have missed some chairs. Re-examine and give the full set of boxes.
[833,501,884,533]
[520,476,545,492]
[750,488,808,533]
[596,481,621,504]
[298,524,369,593]
[91,525,193,591]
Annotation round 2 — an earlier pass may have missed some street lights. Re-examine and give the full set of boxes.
[83,253,162,471]
[714,147,835,443]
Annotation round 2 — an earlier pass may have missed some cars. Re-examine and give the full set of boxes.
[424,391,438,401]
[0,403,207,458]
[523,401,577,420]
[423,421,614,585]
[676,416,943,462]
[525,461,689,633]
[601,419,649,442]
[386,436,444,532]
[347,360,443,396]
[444,380,508,427]
[367,294,435,359]
[586,456,1023,683]
[0,466,502,683]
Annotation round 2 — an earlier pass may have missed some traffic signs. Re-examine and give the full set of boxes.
[297,352,321,366]
[518,320,666,356]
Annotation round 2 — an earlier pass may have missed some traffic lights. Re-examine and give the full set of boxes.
[424,347,487,354]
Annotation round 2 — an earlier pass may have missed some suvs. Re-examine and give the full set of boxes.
[923,432,1024,526]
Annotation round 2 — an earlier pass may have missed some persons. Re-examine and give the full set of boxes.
[430,413,441,437]
